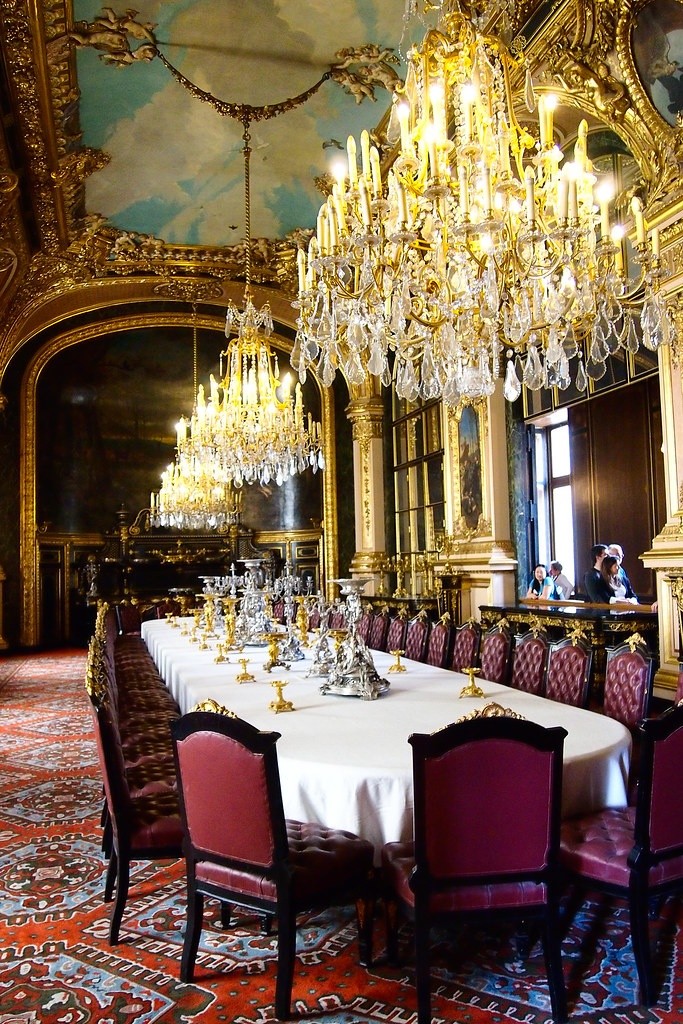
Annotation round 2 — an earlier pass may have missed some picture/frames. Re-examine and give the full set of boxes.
[447,394,493,543]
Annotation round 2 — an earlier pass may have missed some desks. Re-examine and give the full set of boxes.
[479,606,661,698]
[141,615,633,876]
[340,596,444,621]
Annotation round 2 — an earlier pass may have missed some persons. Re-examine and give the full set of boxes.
[651,601,658,613]
[549,561,573,612]
[583,543,635,614]
[526,564,556,611]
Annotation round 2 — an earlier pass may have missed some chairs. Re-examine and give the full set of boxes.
[84,594,240,945]
[380,697,683,1024]
[169,699,379,1022]
[272,595,655,736]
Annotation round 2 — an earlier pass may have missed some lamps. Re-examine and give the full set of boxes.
[290,0,675,402]
[109,228,312,531]
[64,6,405,489]
